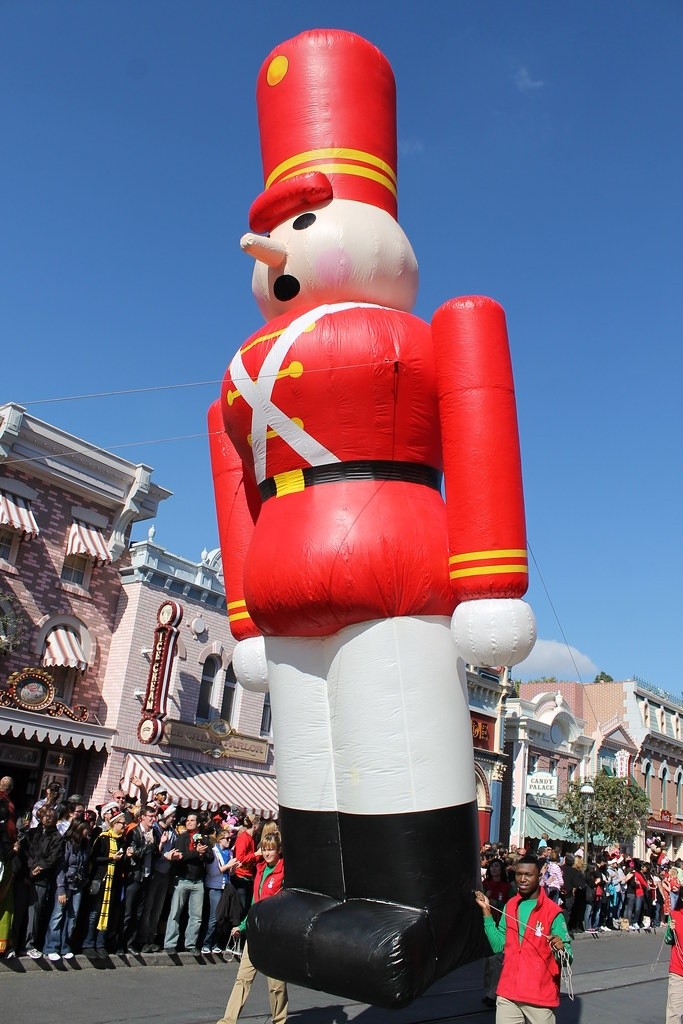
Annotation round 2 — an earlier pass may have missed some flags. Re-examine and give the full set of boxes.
[666,842,675,861]
[675,843,683,862]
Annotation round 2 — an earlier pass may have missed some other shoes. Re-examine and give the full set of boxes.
[82,947,96,958]
[44,951,61,961]
[4,950,15,961]
[585,927,599,933]
[97,947,109,959]
[61,951,74,959]
[140,942,161,952]
[166,947,177,955]
[201,945,211,953]
[600,925,612,931]
[127,948,141,957]
[188,947,200,956]
[21,947,42,959]
[212,946,222,954]
[629,923,640,930]
[115,948,124,957]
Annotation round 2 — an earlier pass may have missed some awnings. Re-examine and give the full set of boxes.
[121,753,279,819]
[524,806,613,847]
[0,707,115,754]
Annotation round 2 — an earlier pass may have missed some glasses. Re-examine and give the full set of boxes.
[114,796,125,800]
[117,821,126,824]
[220,837,231,840]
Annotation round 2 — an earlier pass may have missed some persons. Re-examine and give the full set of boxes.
[474,855,573,1024]
[482,859,514,927]
[664,886,683,1024]
[0,775,280,959]
[216,833,285,1024]
[479,833,683,934]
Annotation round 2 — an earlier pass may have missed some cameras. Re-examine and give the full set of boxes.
[51,785,61,792]
[118,848,126,854]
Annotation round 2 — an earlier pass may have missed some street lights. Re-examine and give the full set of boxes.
[579,784,596,872]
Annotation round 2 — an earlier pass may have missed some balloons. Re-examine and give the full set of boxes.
[209,27,538,1007]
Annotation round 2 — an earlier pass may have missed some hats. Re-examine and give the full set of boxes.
[96,802,120,820]
[154,787,166,794]
[157,804,175,820]
[109,811,124,823]
